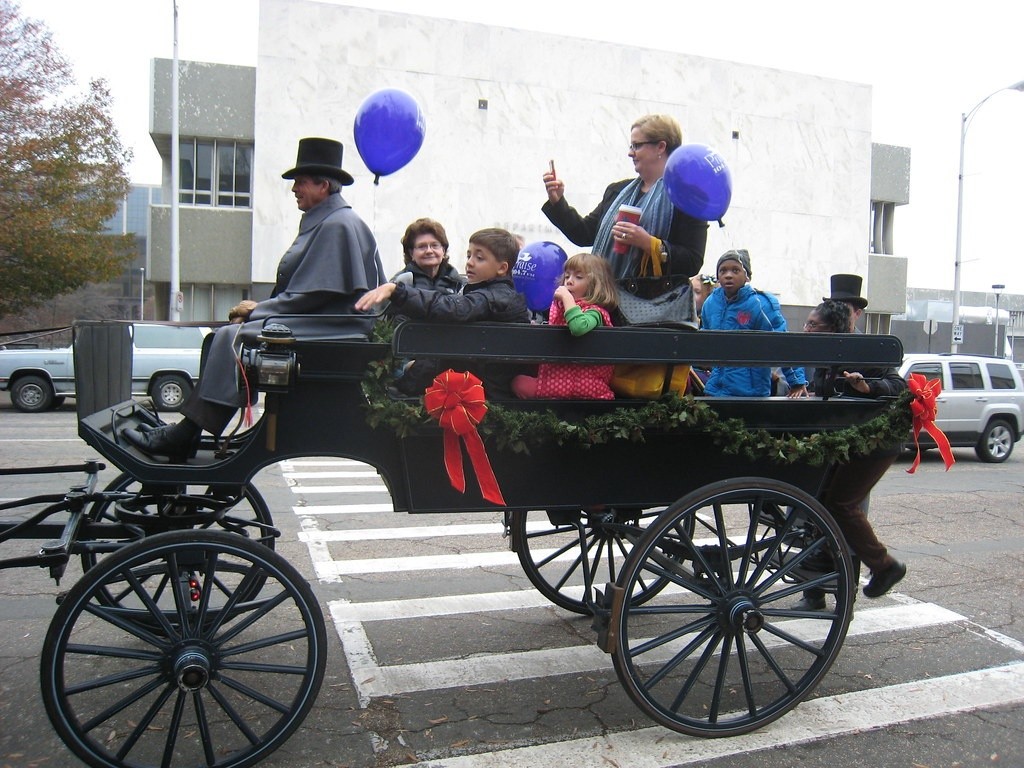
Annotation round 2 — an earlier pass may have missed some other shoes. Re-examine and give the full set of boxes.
[790,594,826,611]
[863,558,906,598]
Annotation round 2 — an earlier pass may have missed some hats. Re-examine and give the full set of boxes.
[282,137,354,186]
[716,249,752,278]
[822,274,868,310]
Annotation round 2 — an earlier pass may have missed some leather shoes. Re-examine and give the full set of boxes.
[121,423,201,463]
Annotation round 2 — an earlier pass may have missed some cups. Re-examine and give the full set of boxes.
[612,202,643,255]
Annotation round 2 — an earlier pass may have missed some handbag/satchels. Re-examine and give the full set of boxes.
[608,239,699,331]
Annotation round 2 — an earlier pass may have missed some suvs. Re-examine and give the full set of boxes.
[0,319,213,415]
[893,352,1024,463]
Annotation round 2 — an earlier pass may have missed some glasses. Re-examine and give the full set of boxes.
[414,241,444,250]
[803,324,832,331]
[629,141,657,152]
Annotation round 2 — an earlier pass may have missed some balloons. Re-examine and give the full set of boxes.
[354,89,426,185]
[511,241,568,320]
[663,144,732,228]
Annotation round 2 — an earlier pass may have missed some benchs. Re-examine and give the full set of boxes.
[393,319,904,434]
[227,299,395,456]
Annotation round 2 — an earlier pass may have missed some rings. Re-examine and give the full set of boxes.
[622,233,625,239]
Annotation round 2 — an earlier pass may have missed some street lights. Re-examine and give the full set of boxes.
[991,284,1006,357]
[1010,314,1018,362]
[949,80,1024,359]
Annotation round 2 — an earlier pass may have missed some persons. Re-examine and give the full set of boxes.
[773,274,907,611]
[512,253,620,400]
[689,274,720,316]
[119,138,388,463]
[352,229,534,397]
[541,117,708,327]
[389,217,467,295]
[700,248,811,399]
